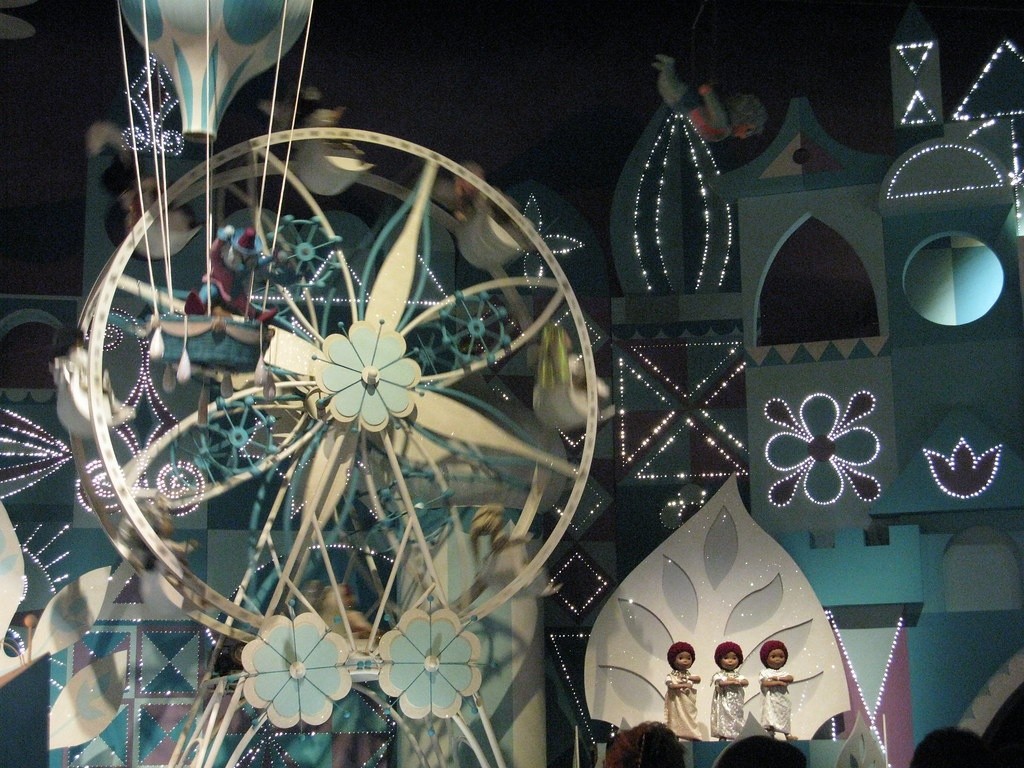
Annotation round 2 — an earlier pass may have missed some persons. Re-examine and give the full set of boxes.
[710,642,749,742]
[760,641,793,741]
[664,642,709,742]
